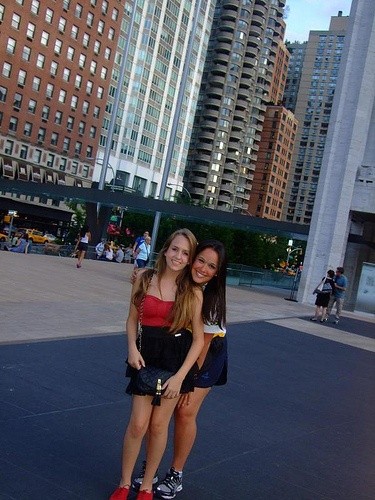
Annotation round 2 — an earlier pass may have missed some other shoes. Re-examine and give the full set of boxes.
[137,489,153,500]
[310,318,317,322]
[110,485,131,500]
[4,245,8,251]
[77,263,82,268]
[334,320,339,324]
[320,320,324,323]
[321,317,328,320]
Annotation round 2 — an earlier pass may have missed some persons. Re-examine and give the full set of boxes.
[130,241,229,500]
[108,228,204,500]
[96,231,152,271]
[4,235,33,255]
[74,224,91,268]
[310,267,349,325]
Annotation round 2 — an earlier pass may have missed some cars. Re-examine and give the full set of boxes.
[15,228,57,244]
[0,233,9,242]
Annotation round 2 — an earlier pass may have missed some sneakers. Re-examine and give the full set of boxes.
[131,460,158,490]
[154,467,183,499]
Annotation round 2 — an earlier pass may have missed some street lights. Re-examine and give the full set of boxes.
[88,158,116,192]
[167,183,192,205]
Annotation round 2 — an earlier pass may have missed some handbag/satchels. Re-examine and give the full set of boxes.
[146,257,149,264]
[138,366,172,406]
[322,283,332,293]
[134,259,138,269]
[316,277,326,293]
